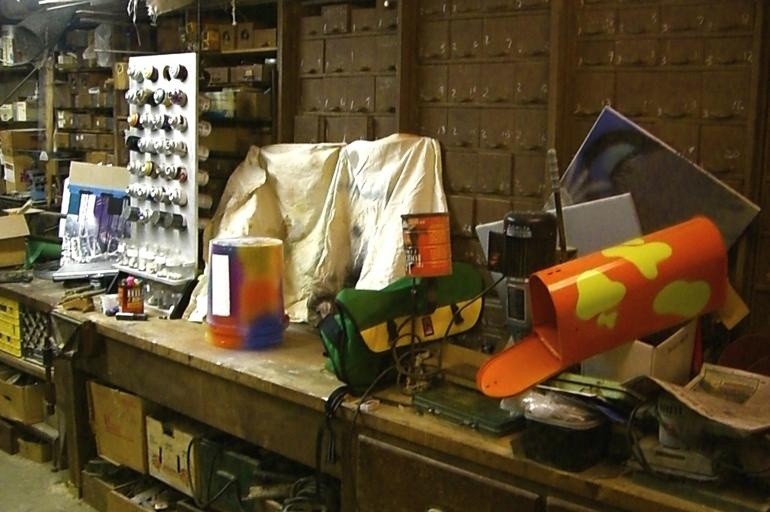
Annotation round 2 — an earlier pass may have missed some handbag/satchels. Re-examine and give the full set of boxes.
[320,263,483,392]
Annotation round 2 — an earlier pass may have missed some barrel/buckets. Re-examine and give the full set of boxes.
[206,238,285,348]
[401,213,454,277]
